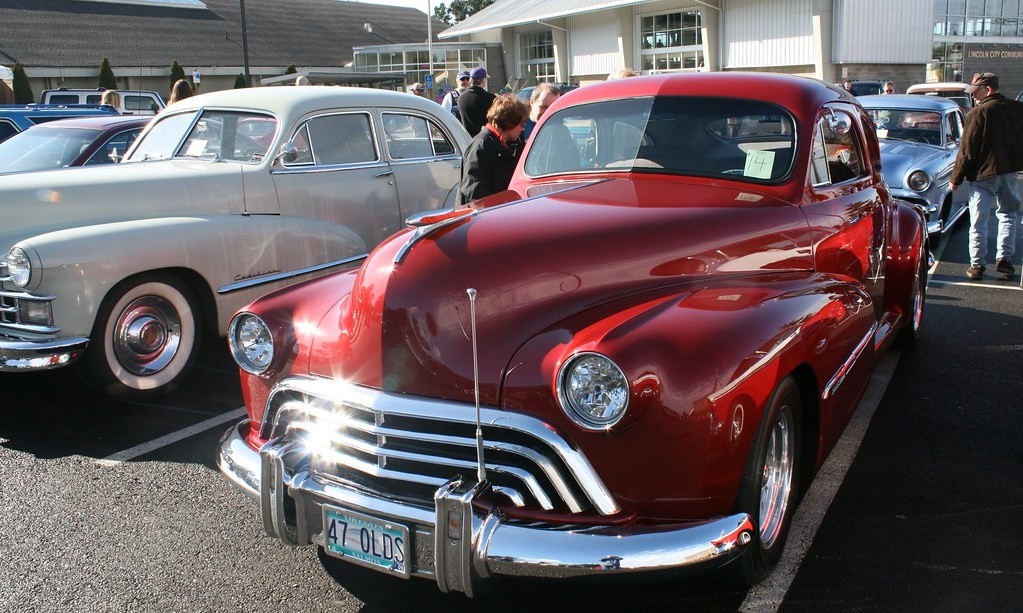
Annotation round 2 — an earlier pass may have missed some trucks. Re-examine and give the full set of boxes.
[40,87,167,116]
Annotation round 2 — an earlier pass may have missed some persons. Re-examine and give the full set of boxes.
[842,79,858,98]
[949,73,1023,279]
[99,90,121,112]
[590,68,638,163]
[874,82,907,128]
[442,70,472,127]
[408,83,433,139]
[519,82,578,174]
[167,79,195,107]
[294,76,309,86]
[458,67,498,138]
[455,94,529,211]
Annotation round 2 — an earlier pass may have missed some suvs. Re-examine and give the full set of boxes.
[0,102,121,147]
[846,80,891,96]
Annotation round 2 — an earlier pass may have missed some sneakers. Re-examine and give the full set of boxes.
[967,264,985,280]
[995,259,1015,275]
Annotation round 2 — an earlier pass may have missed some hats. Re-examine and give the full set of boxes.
[964,73,999,94]
[412,83,424,90]
[457,69,470,79]
[296,76,309,86]
[470,67,491,79]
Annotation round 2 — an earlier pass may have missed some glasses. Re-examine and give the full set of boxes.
[886,90,893,92]
[520,122,526,127]
[461,78,469,81]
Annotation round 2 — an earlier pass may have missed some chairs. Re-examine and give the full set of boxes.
[321,139,374,161]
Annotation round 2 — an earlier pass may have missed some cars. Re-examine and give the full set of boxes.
[0,83,471,401]
[852,83,972,237]
[0,116,310,176]
[214,71,926,592]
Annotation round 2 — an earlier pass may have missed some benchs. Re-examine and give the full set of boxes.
[887,128,939,141]
[629,138,851,183]
